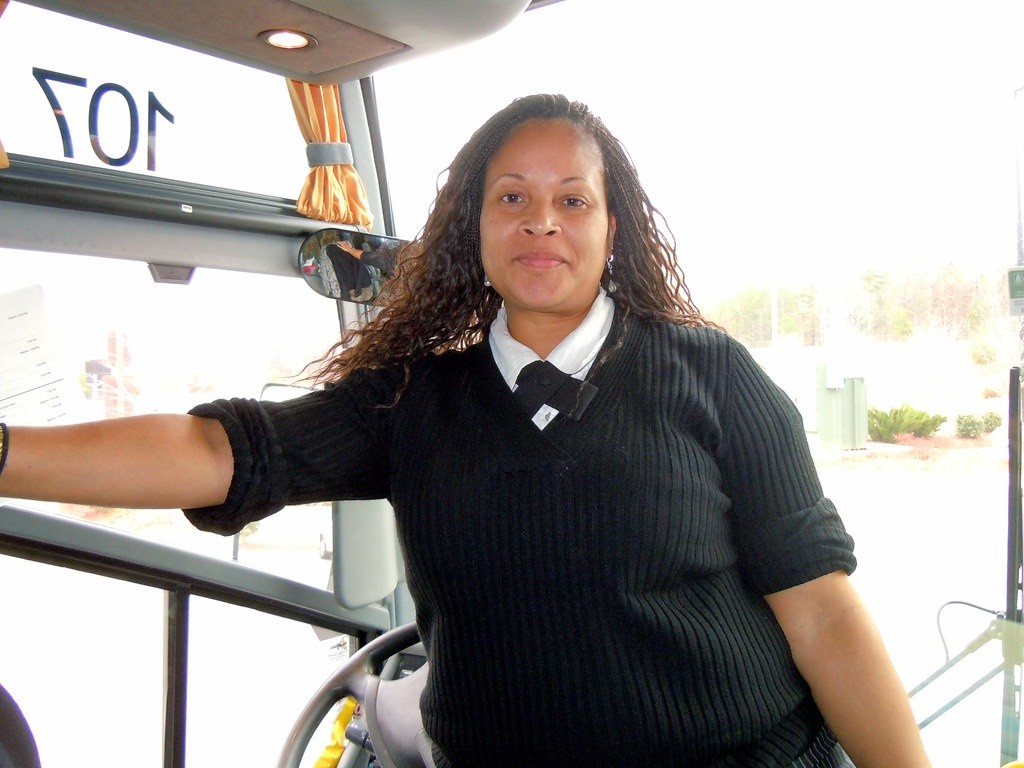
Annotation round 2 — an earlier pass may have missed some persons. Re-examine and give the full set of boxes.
[337,237,409,303]
[0,94,935,768]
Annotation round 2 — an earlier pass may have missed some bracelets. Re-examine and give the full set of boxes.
[0,423,9,474]
